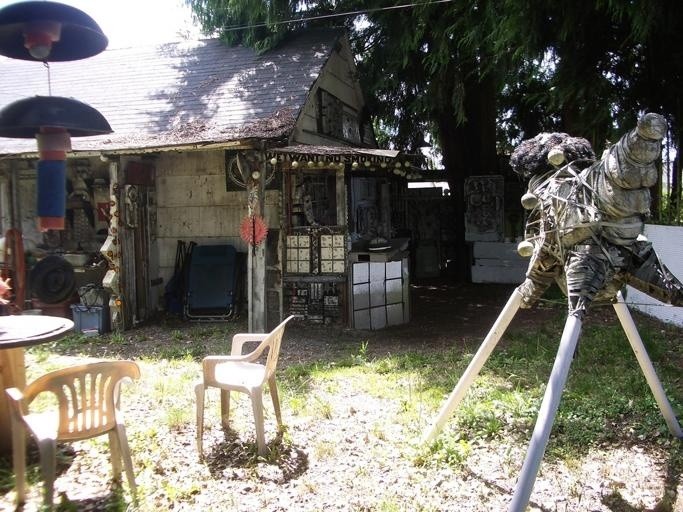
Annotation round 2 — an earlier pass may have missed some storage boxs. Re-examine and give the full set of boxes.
[31,251,111,336]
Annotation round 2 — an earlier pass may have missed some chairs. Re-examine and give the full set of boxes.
[178,245,241,324]
[189,314,295,464]
[4,358,143,510]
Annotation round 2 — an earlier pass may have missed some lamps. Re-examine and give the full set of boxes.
[0,0,114,233]
[248,148,424,182]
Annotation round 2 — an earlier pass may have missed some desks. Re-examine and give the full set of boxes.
[0,313,76,464]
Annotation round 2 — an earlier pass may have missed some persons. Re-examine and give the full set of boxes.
[0,230,15,298]
[468,180,502,226]
